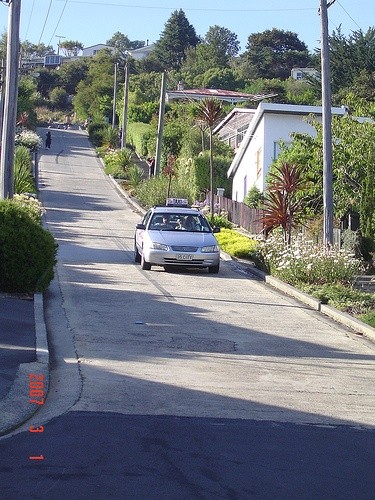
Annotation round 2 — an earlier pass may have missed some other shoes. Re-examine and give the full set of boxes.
[44,147,50,151]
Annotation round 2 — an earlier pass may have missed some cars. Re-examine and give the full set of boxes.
[134,197,222,274]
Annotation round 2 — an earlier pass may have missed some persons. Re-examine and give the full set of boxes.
[45,131,51,150]
[153,213,206,231]
